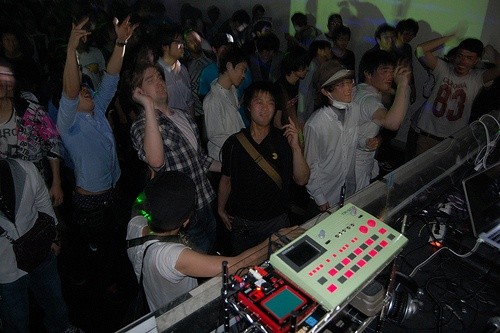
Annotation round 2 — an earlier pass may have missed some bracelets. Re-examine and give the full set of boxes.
[116,40,127,45]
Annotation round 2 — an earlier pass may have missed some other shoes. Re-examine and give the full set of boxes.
[64,323,85,333]
[378,160,393,171]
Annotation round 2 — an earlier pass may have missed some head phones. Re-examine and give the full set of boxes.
[386,284,423,322]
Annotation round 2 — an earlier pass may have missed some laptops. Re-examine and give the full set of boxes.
[463,161,500,250]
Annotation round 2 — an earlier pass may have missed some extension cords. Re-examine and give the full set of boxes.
[428,203,451,248]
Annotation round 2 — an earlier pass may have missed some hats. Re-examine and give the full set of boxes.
[146,169,195,229]
[317,59,353,92]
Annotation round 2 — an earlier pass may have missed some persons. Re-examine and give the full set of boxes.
[126,170,304,312]
[411,35,500,155]
[0,0,418,333]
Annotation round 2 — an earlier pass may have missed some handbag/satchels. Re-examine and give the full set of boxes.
[13,211,56,272]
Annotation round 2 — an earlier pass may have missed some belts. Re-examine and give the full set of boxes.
[419,130,444,142]
